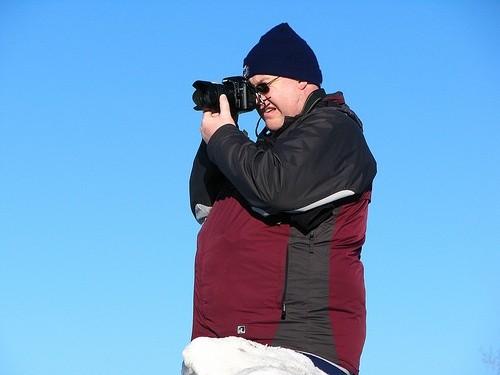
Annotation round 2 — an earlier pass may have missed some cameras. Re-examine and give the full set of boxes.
[192,76,256,114]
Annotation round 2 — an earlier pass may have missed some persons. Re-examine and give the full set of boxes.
[188,22,377,375]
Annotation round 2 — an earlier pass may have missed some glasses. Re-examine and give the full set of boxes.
[255,76,280,94]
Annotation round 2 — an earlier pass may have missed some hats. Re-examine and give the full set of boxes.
[243,21,323,84]
